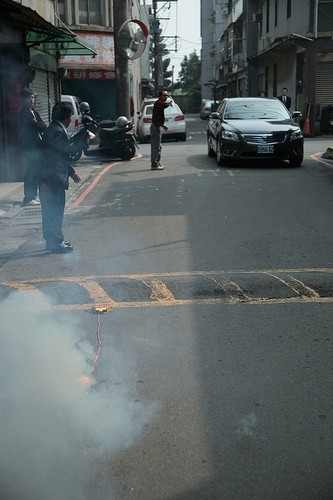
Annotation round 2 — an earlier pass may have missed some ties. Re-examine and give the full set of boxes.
[284,96,285,103]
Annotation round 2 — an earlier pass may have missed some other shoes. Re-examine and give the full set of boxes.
[23,197,40,205]
[46,240,74,252]
[151,164,165,170]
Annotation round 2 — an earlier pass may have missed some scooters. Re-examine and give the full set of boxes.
[68,109,140,162]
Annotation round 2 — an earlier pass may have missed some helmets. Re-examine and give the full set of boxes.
[115,116,127,126]
[79,102,90,112]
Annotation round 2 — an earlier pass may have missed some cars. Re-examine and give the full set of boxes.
[137,95,188,144]
[206,96,305,169]
[200,98,222,120]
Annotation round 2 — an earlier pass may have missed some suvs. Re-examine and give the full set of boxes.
[61,95,84,134]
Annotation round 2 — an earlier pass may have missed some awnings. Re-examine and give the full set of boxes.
[28,32,98,59]
[0,0,77,48]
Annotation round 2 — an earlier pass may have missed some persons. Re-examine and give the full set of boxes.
[19,88,47,206]
[39,102,81,253]
[277,88,291,110]
[150,90,172,170]
[259,90,266,97]
